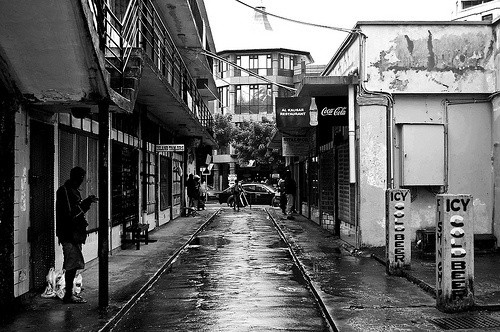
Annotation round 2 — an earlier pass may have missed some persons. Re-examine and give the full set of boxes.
[278,171,298,220]
[56,165,97,303]
[232,179,242,210]
[185,174,206,215]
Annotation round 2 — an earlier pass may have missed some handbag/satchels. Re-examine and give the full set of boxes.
[40,267,82,298]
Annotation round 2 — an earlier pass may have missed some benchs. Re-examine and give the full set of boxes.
[121,206,150,250]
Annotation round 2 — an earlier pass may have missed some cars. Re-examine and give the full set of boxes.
[195,183,281,207]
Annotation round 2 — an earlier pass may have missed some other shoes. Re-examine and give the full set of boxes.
[62,294,88,304]
